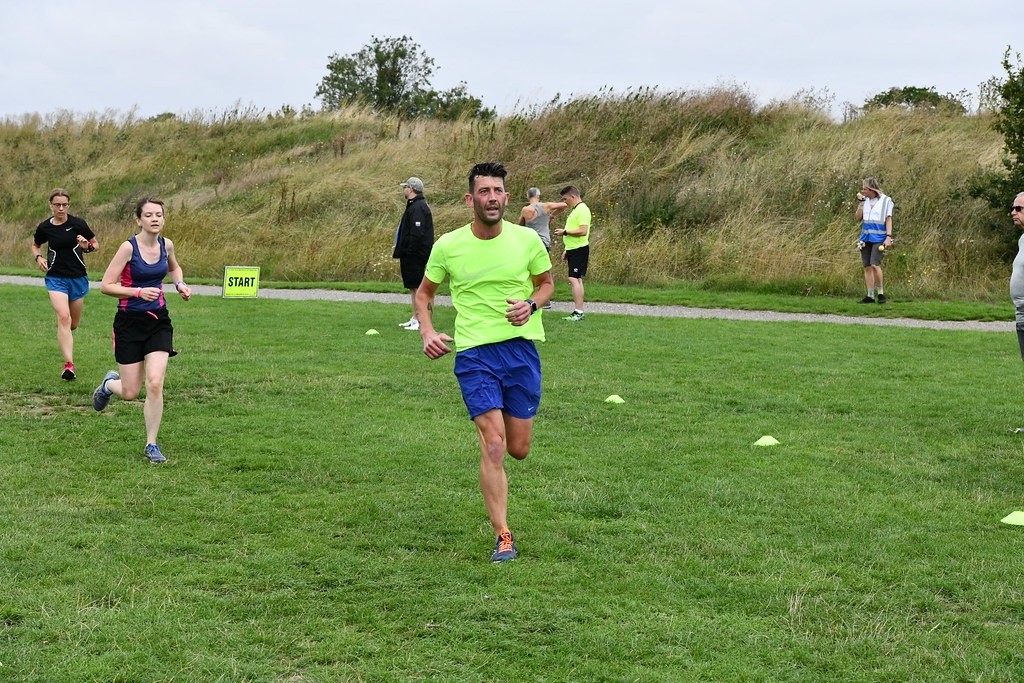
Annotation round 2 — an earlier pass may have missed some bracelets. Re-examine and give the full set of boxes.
[563,230,567,235]
[887,234,892,237]
[176,281,186,291]
[34,254,42,262]
[82,241,93,253]
[135,287,141,298]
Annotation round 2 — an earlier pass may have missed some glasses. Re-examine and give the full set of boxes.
[1012,205,1024,212]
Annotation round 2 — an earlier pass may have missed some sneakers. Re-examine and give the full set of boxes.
[61,362,76,379]
[562,310,585,320]
[491,525,518,562]
[143,443,166,463]
[93,370,119,411]
[405,320,420,330]
[398,316,417,326]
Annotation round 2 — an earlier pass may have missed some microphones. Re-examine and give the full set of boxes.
[878,239,894,251]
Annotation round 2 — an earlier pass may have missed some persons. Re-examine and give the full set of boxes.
[518,187,567,309]
[32,188,99,380]
[415,162,554,563]
[555,185,591,321]
[92,197,192,463]
[392,176,434,330]
[855,177,895,304]
[1010,192,1024,360]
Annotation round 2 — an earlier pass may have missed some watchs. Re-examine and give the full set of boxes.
[525,299,537,312]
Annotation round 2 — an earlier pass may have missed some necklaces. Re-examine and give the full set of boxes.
[574,201,582,208]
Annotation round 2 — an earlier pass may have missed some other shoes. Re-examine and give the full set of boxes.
[542,301,551,308]
[878,294,886,303]
[858,296,875,303]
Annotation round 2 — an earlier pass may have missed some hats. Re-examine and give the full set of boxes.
[400,176,423,192]
